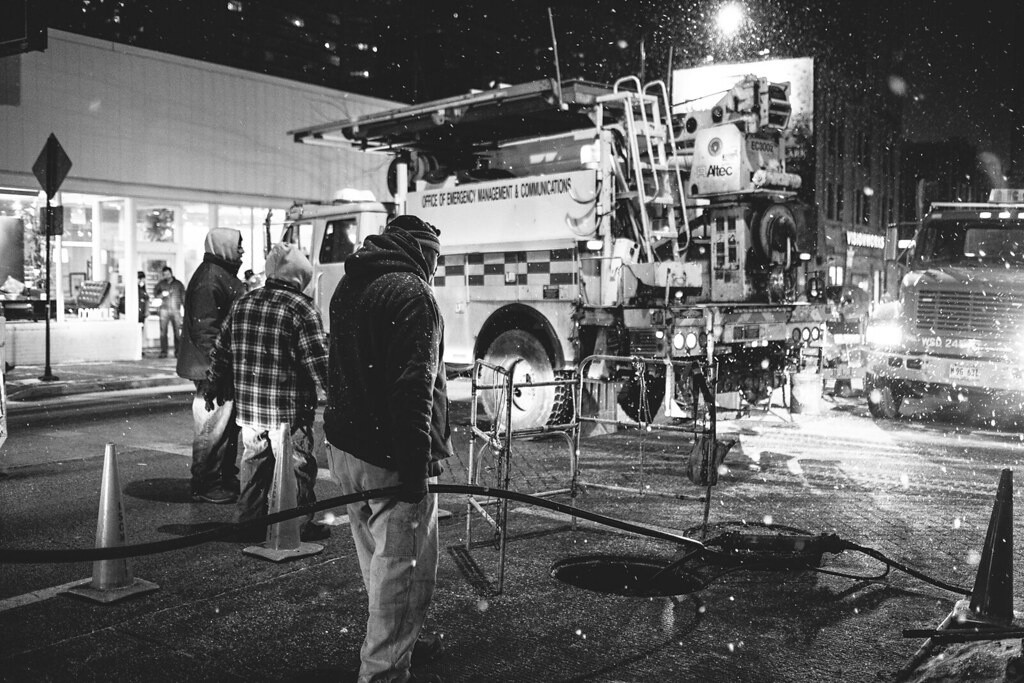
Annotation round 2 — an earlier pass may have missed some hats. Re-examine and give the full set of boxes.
[138,271,145,279]
[382,215,440,255]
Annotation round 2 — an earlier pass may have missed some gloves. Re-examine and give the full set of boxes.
[202,381,225,413]
[399,477,429,505]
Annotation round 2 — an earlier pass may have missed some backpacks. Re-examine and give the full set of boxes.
[616,362,667,425]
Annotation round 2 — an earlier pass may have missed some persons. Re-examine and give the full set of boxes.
[324,216,451,683]
[203,242,331,540]
[115,267,259,358]
[175,228,246,501]
[320,223,359,263]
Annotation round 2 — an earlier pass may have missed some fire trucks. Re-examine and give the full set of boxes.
[860,187,1023,428]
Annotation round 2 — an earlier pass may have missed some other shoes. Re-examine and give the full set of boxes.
[175,352,179,358]
[238,527,266,543]
[411,633,442,657]
[191,486,239,503]
[300,520,331,540]
[406,673,441,683]
[159,352,168,358]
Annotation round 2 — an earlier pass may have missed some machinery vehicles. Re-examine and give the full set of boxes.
[273,0,819,441]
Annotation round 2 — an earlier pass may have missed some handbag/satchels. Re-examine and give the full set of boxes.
[685,430,736,487]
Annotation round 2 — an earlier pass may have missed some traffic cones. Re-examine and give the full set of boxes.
[241,421,325,562]
[66,441,162,604]
[955,466,1016,629]
